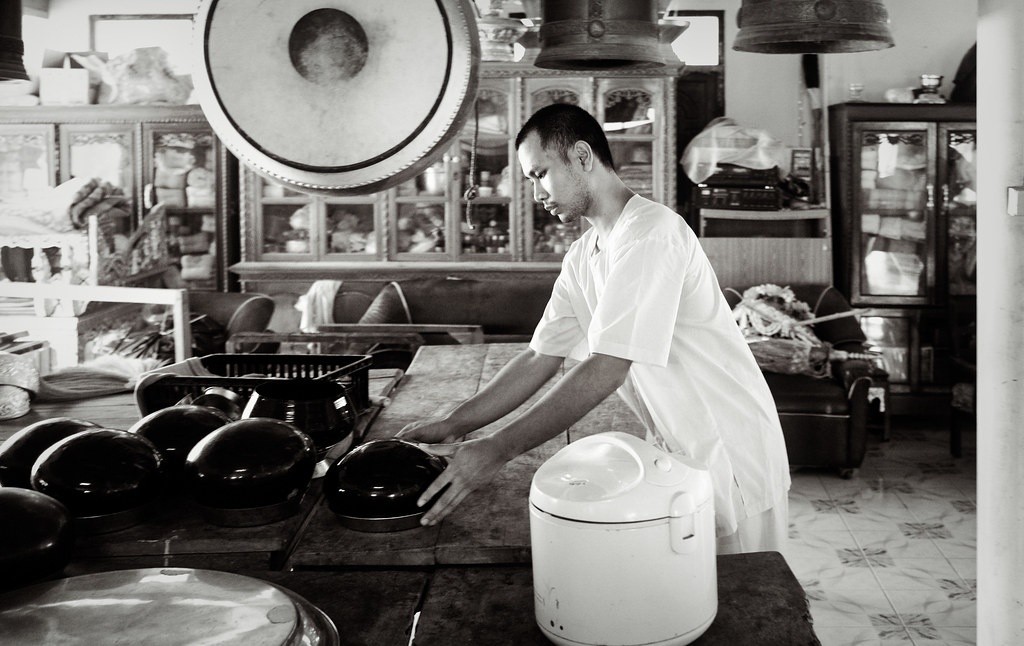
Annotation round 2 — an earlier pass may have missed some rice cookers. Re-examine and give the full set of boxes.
[527,430,719,646]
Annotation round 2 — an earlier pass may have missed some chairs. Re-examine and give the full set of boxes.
[190,289,276,355]
[947,374,977,456]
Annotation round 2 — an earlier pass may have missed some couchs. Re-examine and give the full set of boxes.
[727,281,875,481]
[319,274,560,345]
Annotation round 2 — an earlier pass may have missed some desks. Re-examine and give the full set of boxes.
[0,340,822,646]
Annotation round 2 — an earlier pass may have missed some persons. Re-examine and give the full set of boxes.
[391,102,791,558]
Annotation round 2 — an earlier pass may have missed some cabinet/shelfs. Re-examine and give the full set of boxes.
[701,207,833,293]
[1,103,230,294]
[227,40,680,334]
[824,102,977,430]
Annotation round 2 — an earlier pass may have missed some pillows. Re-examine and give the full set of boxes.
[352,281,424,349]
